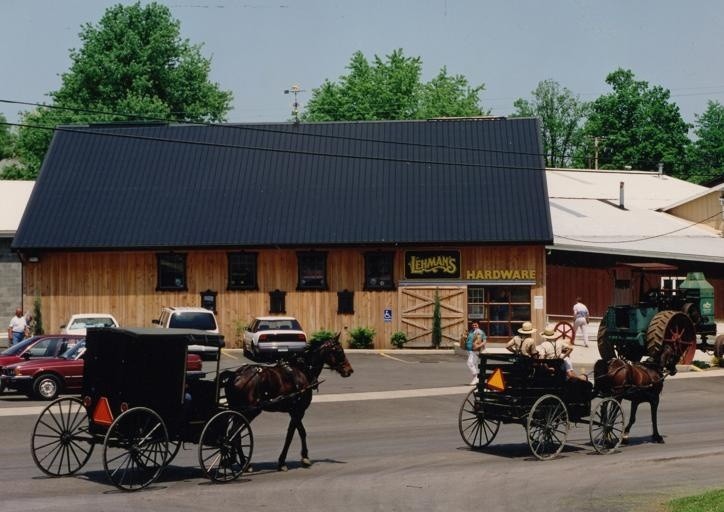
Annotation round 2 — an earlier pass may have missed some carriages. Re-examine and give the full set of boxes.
[30,326,355,492]
[456,343,676,463]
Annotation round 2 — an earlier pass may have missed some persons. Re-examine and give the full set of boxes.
[572,297,590,348]
[538,327,573,360]
[463,321,487,386]
[505,322,540,359]
[8,309,30,345]
[560,348,586,384]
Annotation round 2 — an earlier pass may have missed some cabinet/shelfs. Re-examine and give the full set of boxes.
[468,287,485,320]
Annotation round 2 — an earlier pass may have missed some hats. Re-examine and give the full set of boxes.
[539,324,563,340]
[517,321,538,334]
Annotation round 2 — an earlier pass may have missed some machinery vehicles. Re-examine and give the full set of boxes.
[598,271,724,369]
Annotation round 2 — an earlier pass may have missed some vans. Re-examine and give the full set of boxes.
[149,306,221,362]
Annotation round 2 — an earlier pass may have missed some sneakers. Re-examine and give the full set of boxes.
[470,378,479,385]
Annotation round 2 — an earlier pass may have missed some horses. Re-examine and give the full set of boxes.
[215,330,354,472]
[592,343,677,447]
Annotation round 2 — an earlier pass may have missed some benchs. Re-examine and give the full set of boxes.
[475,353,564,414]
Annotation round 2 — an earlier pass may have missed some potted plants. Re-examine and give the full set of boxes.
[432,285,442,349]
[355,343,361,349]
[346,325,377,349]
[390,332,408,349]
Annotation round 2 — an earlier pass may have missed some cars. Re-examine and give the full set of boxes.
[58,313,119,334]
[0,335,204,401]
[241,316,308,359]
